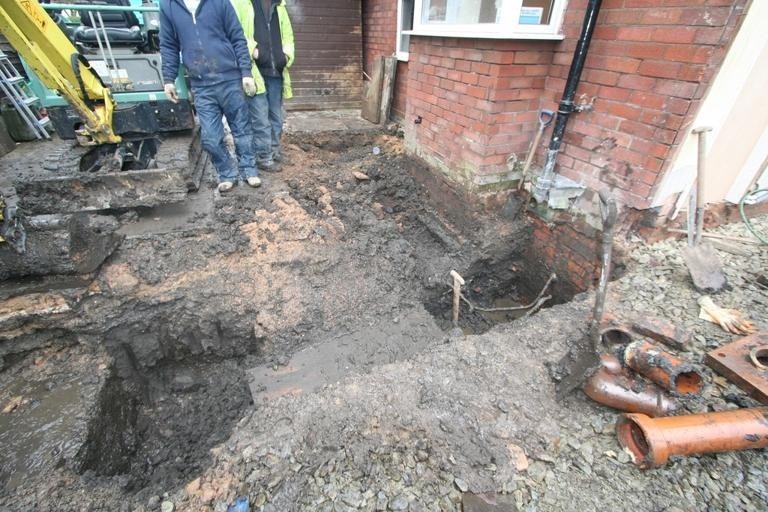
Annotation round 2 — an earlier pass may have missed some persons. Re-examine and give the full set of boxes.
[159,1,295,192]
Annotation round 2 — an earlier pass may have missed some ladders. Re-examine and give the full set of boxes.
[0,48,57,141]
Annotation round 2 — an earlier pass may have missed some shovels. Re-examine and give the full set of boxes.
[555,187,618,404]
[498,108,555,222]
[682,127,727,294]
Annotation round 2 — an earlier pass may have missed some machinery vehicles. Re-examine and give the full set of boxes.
[0,0,205,279]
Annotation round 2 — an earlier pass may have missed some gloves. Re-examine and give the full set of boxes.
[242,77,256,98]
[164,83,178,105]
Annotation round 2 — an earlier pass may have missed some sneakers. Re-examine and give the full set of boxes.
[258,153,287,173]
[217,181,232,192]
[247,177,262,188]
